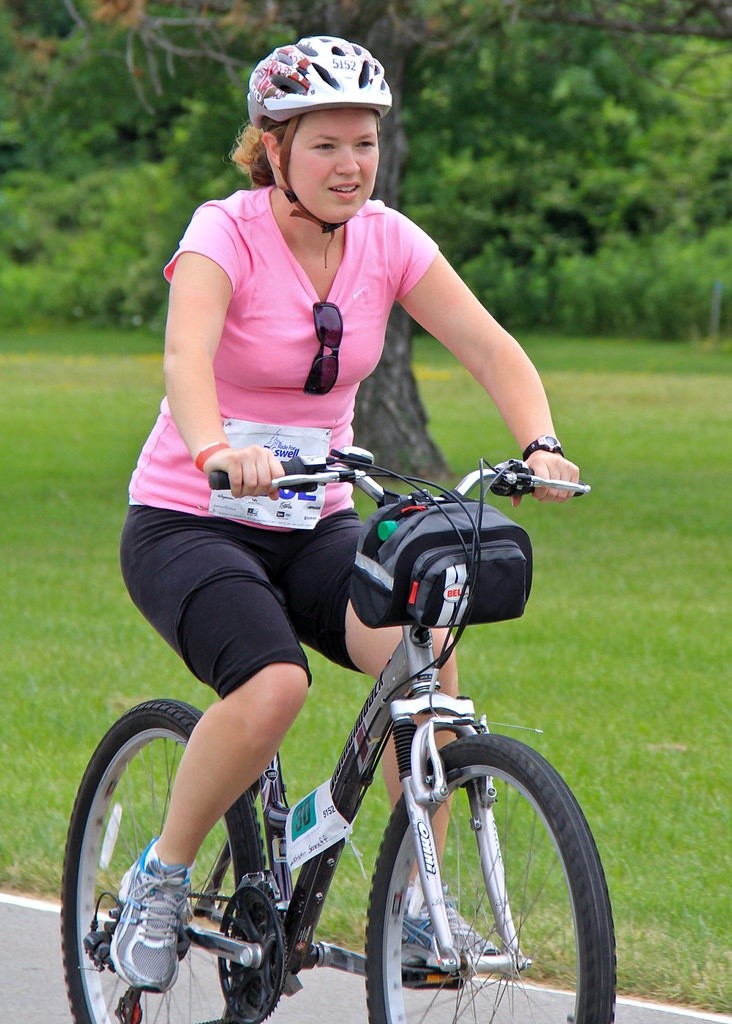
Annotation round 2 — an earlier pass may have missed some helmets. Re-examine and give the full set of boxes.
[248,37,394,120]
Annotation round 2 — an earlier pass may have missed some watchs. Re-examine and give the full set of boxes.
[518,432,567,463]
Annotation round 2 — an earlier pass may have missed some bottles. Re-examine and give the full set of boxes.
[378,515,410,541]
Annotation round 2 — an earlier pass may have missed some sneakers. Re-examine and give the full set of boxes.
[390,884,503,968]
[108,838,191,991]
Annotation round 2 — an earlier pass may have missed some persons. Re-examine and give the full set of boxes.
[106,34,584,1003]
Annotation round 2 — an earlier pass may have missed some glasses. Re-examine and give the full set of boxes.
[303,304,344,395]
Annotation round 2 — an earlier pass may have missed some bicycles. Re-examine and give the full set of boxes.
[62,446,618,1024]
